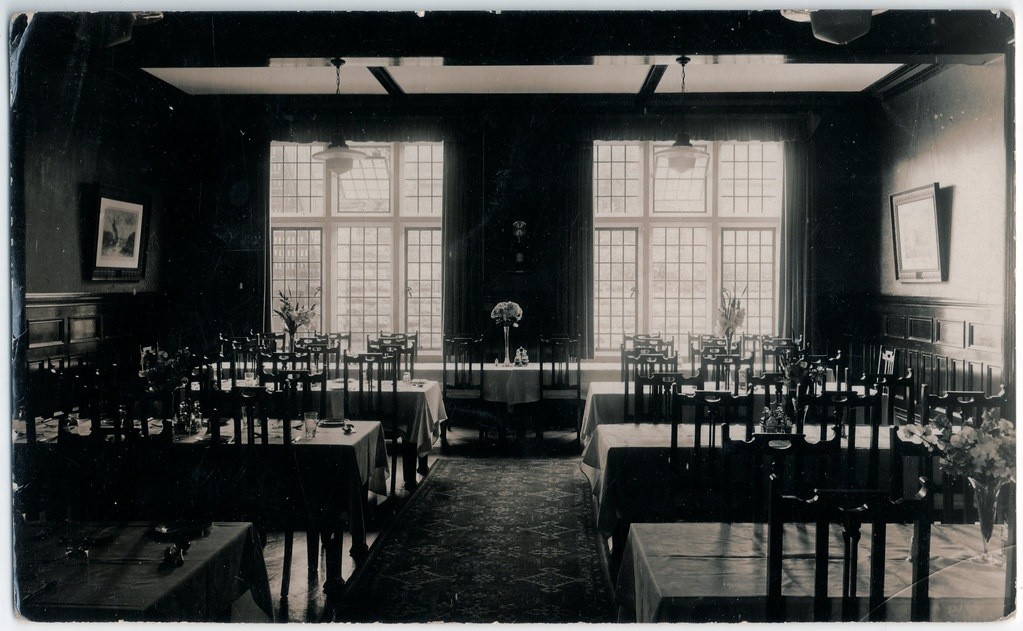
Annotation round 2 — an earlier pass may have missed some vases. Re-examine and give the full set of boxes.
[792,384,815,435]
[502,324,513,368]
[288,332,296,353]
[726,336,734,355]
[967,477,1001,562]
[155,389,175,437]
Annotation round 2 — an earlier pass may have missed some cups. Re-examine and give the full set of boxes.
[304,412,318,440]
[403,372,411,389]
[245,372,254,387]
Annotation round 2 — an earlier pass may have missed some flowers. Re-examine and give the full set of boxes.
[271,288,318,332]
[903,404,1016,501]
[491,301,522,322]
[139,342,196,403]
[776,332,827,396]
[712,287,747,336]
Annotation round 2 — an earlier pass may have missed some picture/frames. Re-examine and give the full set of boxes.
[889,183,946,284]
[84,182,151,283]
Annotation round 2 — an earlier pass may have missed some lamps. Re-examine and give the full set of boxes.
[781,9,885,46]
[311,57,366,174]
[653,58,711,174]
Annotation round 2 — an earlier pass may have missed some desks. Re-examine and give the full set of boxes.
[581,382,882,454]
[582,424,991,526]
[15,411,392,591]
[14,518,276,622]
[615,522,1014,623]
[211,361,834,449]
[192,377,449,488]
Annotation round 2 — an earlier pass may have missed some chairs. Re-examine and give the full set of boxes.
[442,337,494,442]
[619,335,1010,620]
[12,331,419,599]
[537,335,581,439]
